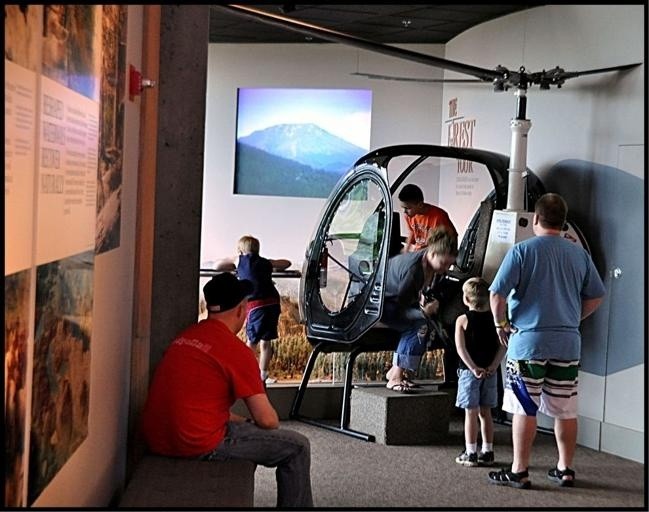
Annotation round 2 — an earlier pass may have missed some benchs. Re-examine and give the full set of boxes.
[118,455,258,508]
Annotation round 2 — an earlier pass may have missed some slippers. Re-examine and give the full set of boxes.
[385,383,417,395]
[402,378,418,388]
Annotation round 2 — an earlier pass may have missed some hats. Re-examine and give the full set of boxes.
[202,272,255,315]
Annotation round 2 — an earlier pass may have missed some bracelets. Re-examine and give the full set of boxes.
[493,319,509,329]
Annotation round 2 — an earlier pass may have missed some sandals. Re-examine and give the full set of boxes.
[487,463,531,490]
[547,467,575,487]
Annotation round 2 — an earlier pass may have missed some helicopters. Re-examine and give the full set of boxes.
[217,0,644,444]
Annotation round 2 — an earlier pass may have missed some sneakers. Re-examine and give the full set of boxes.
[477,451,494,466]
[455,450,478,467]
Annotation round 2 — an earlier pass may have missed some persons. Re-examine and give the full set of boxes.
[487,191,607,489]
[141,273,315,508]
[454,276,505,466]
[213,235,292,381]
[398,183,458,255]
[373,225,459,392]
